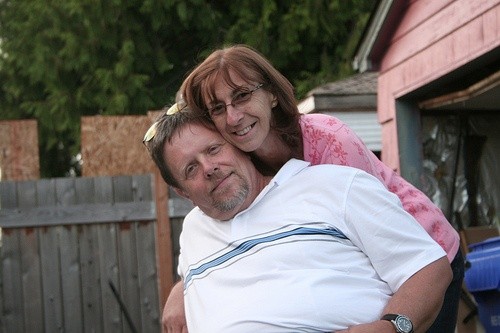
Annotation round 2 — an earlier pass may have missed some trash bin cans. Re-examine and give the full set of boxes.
[463,236,500,333]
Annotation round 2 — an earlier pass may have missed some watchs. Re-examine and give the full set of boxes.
[380,312,414,332]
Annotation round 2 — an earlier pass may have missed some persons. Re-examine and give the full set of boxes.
[161,42,464,333]
[141,102,453,332]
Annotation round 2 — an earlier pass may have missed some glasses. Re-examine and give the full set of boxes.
[143,100,188,153]
[208,83,263,115]
